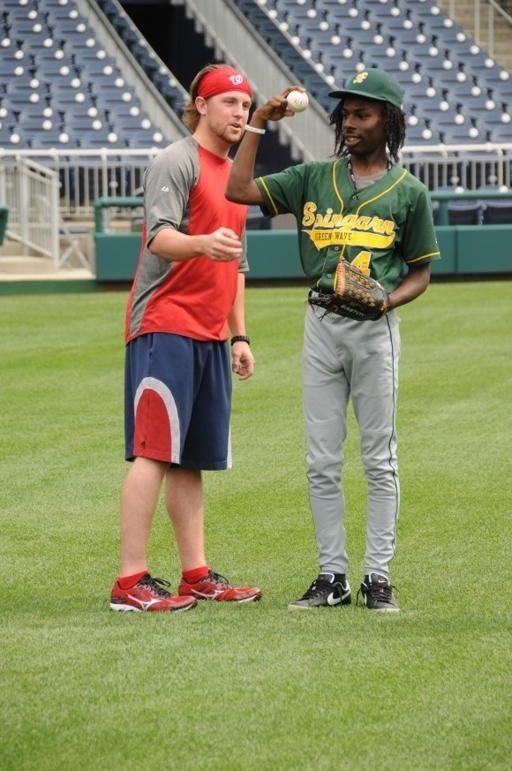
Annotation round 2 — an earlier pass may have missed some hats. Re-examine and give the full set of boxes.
[329,68,406,109]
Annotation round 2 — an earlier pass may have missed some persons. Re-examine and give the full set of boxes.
[111,64,263,614]
[224,69,441,614]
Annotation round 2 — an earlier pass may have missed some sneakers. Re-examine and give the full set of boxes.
[109,571,402,614]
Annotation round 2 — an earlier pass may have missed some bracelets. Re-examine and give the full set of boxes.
[230,335,250,345]
[244,125,265,135]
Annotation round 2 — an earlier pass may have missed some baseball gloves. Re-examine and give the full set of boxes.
[308,256,388,321]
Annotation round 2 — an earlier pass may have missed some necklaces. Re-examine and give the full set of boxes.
[348,158,392,190]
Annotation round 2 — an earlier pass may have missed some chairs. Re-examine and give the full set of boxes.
[241,5,511,180]
[2,3,176,170]
[429,194,512,229]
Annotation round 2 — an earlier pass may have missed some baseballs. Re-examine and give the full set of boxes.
[288,90,307,112]
